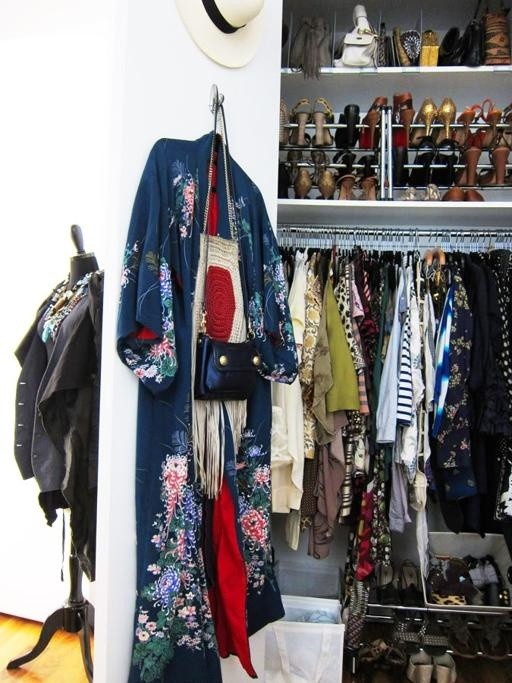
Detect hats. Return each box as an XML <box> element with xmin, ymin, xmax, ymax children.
<box><xmin>174</xmin><ymin>1</ymin><xmax>270</xmax><ymax>70</ymax></box>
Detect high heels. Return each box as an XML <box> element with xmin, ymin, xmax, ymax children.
<box><xmin>409</xmin><ymin>97</ymin><xmax>456</xmax><ymax>149</ymax></box>
<box><xmin>393</xmin><ymin>145</ymin><xmax>410</xmax><ymax>187</ymax></box>
<box><xmin>451</xmin><ymin>100</ymin><xmax>502</xmax><ymax>149</ymax></box>
<box><xmin>288</xmin><ymin>96</ymin><xmax>334</xmax><ymax>148</ymax></box>
<box><xmin>335</xmin><ymin>173</ymin><xmax>378</xmax><ymax>199</ymax></box>
<box><xmin>293</xmin><ymin>168</ymin><xmax>335</xmax><ymax>199</ymax></box>
<box><xmin>453</xmin><ymin>132</ymin><xmax>511</xmax><ymax>187</ymax></box>
<box><xmin>406</xmin><ymin>652</ymin><xmax>458</xmax><ymax>683</ymax></box>
<box><xmin>356</xmin><ymin>637</ymin><xmax>406</xmax><ymax>683</ymax></box>
<box><xmin>359</xmin><ymin>92</ymin><xmax>416</xmax><ymax>152</ymax></box>
<box><xmin>408</xmin><ymin>136</ymin><xmax>459</xmax><ymax>188</ymax></box>
<box><xmin>334</xmin><ymin>103</ymin><xmax>360</xmax><ymax>148</ymax></box>
<box><xmin>333</xmin><ymin>150</ymin><xmax>378</xmax><ymax>185</ymax></box>
<box><xmin>287</xmin><ymin>151</ymin><xmax>331</xmax><ymax>186</ymax></box>
<box><xmin>279</xmin><ymin>161</ymin><xmax>291</xmax><ymax>199</ymax></box>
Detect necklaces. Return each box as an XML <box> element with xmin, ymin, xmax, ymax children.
<box><xmin>41</xmin><ymin>271</ymin><xmax>94</xmax><ymax>342</ymax></box>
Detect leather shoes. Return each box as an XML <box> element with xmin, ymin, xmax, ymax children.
<box><xmin>428</xmin><ymin>568</ymin><xmax>467</xmax><ymax>605</ymax></box>
<box><xmin>279</xmin><ymin>97</ymin><xmax>290</xmax><ymax>146</ymax></box>
<box><xmin>375</xmin><ymin>558</ymin><xmax>424</xmax><ymax>607</ymax></box>
<box><xmin>463</xmin><ymin>554</ymin><xmax>510</xmax><ymax>607</ymax></box>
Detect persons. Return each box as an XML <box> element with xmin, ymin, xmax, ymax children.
<box><xmin>11</xmin><ymin>250</ymin><xmax>104</xmax><ymax>584</ymax></box>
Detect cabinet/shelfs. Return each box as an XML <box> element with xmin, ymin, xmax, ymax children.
<box><xmin>266</xmin><ymin>0</ymin><xmax>509</xmax><ymax>622</ymax></box>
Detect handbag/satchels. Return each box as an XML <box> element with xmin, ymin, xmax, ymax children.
<box><xmin>288</xmin><ymin>13</ymin><xmax>336</xmax><ymax>81</ymax></box>
<box><xmin>193</xmin><ymin>332</ymin><xmax>262</xmax><ymax>402</ymax></box>
<box><xmin>340</xmin><ymin>26</ymin><xmax>380</xmax><ymax>69</ymax></box>
<box><xmin>436</xmin><ymin>0</ymin><xmax>512</xmax><ymax>68</ymax></box>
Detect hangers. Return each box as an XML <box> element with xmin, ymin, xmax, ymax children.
<box><xmin>277</xmin><ymin>221</ymin><xmax>512</xmax><ymax>266</ymax></box>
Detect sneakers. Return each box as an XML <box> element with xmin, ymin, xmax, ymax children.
<box><xmin>442</xmin><ymin>187</ymin><xmax>485</xmax><ymax>201</ymax></box>
<box><xmin>397</xmin><ymin>183</ymin><xmax>441</xmax><ymax>200</ymax></box>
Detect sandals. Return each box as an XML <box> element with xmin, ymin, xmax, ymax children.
<box><xmin>448</xmin><ymin>613</ymin><xmax>509</xmax><ymax>660</ymax></box>
<box><xmin>497</xmin><ymin>103</ymin><xmax>512</xmax><ymax>148</ymax></box>
<box><xmin>391</xmin><ymin>609</ymin><xmax>449</xmax><ymax>656</ymax></box>
